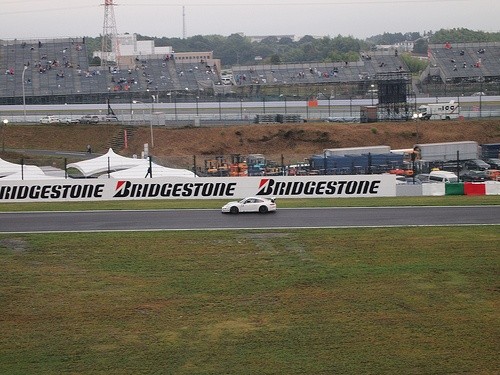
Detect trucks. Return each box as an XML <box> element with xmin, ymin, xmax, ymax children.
<box><xmin>418</xmin><ymin>103</ymin><xmax>460</xmax><ymax>121</ymax></box>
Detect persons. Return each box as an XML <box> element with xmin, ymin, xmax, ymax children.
<box><xmin>0</xmin><ymin>34</ymin><xmax>500</xmax><ymax>91</ymax></box>
<box><xmin>86</xmin><ymin>144</ymin><xmax>92</xmax><ymax>153</ymax></box>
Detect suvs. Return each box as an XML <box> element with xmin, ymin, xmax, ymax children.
<box><xmin>81</xmin><ymin>115</ymin><xmax>99</xmax><ymax>124</ymax></box>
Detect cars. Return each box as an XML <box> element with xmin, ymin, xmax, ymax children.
<box><xmin>221</xmin><ymin>197</ymin><xmax>276</xmax><ymax>214</ymax></box>
<box><xmin>222</xmin><ymin>78</ymin><xmax>230</xmax><ymax>85</ymax></box>
<box><xmin>60</xmin><ymin>117</ymin><xmax>79</xmax><ymax>125</ymax></box>
<box><xmin>202</xmin><ymin>145</ymin><xmax>500</xmax><ymax>188</ymax></box>
<box><xmin>39</xmin><ymin>116</ymin><xmax>59</xmax><ymax>125</ymax></box>
